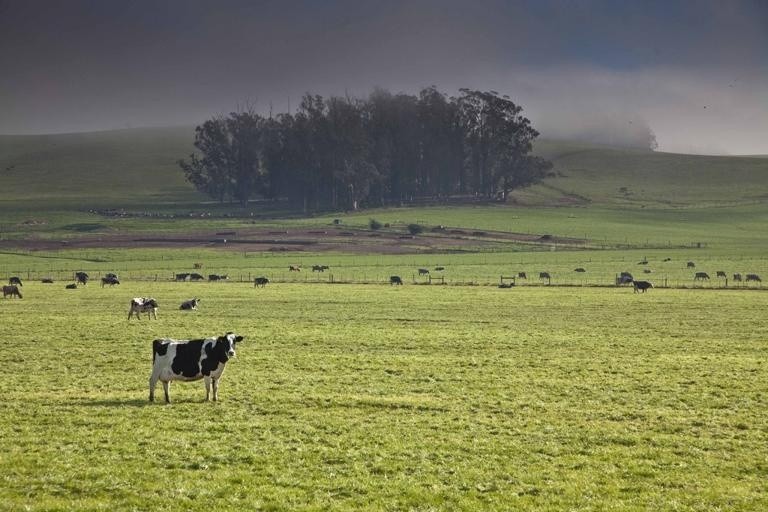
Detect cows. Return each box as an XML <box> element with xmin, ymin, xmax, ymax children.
<box><xmin>3</xmin><ymin>277</ymin><xmax>23</xmax><ymax>298</ymax></box>
<box><xmin>518</xmin><ymin>272</ymin><xmax>526</xmax><ymax>279</ymax></box>
<box><xmin>127</xmin><ymin>297</ymin><xmax>158</xmax><ymax>321</ymax></box>
<box><xmin>539</xmin><ymin>273</ymin><xmax>550</xmax><ymax>280</ymax></box>
<box><xmin>65</xmin><ymin>272</ymin><xmax>89</xmax><ymax>289</ymax></box>
<box><xmin>616</xmin><ymin>272</ymin><xmax>653</xmax><ymax>293</ymax></box>
<box><xmin>99</xmin><ymin>273</ymin><xmax>120</xmax><ymax>288</ymax></box>
<box><xmin>418</xmin><ymin>269</ymin><xmax>430</xmax><ymax>276</ymax></box>
<box><xmin>686</xmin><ymin>260</ymin><xmax>763</xmax><ymax>282</ymax></box>
<box><xmin>175</xmin><ymin>272</ymin><xmax>230</xmax><ymax>283</ymax></box>
<box><xmin>148</xmin><ymin>332</ymin><xmax>244</xmax><ymax>403</ymax></box>
<box><xmin>312</xmin><ymin>264</ymin><xmax>329</xmax><ymax>273</ymax></box>
<box><xmin>179</xmin><ymin>298</ymin><xmax>200</xmax><ymax>310</ymax></box>
<box><xmin>390</xmin><ymin>275</ymin><xmax>403</xmax><ymax>285</ymax></box>
<box><xmin>288</xmin><ymin>265</ymin><xmax>301</xmax><ymax>272</ymax></box>
<box><xmin>253</xmin><ymin>277</ymin><xmax>270</xmax><ymax>289</ymax></box>
